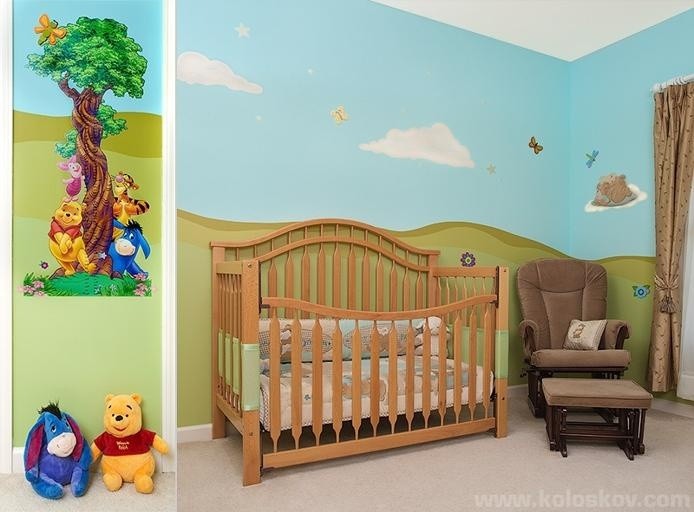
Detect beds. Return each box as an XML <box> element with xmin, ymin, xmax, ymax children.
<box><xmin>206</xmin><ymin>218</ymin><xmax>512</xmax><ymax>490</ymax></box>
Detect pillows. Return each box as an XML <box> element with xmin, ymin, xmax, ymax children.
<box><xmin>562</xmin><ymin>317</ymin><xmax>608</xmax><ymax>351</ymax></box>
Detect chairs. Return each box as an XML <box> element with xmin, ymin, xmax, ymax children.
<box><xmin>513</xmin><ymin>257</ymin><xmax>657</xmax><ymax>461</ymax></box>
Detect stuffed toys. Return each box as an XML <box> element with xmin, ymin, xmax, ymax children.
<box><xmin>22</xmin><ymin>398</ymin><xmax>92</xmax><ymax>502</ymax></box>
<box><xmin>91</xmin><ymin>394</ymin><xmax>172</xmax><ymax>495</ymax></box>
